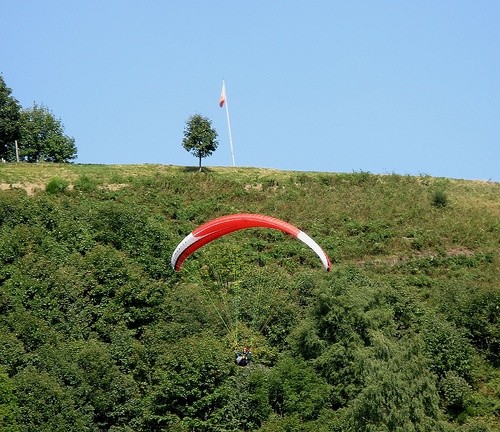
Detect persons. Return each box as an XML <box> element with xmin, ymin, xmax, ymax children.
<box><xmin>240</xmin><ymin>348</ymin><xmax>249</xmax><ymax>360</ymax></box>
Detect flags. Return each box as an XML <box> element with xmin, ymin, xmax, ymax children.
<box><xmin>219</xmin><ymin>89</ymin><xmax>225</xmax><ymax>108</ymax></box>
